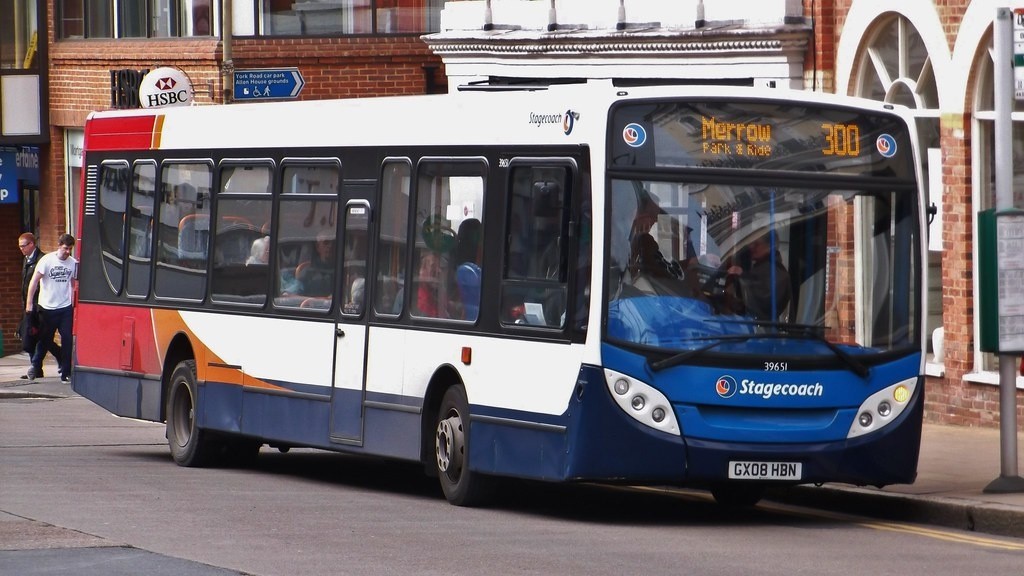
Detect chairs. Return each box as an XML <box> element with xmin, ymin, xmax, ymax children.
<box><xmin>137</xmin><ymin>212</ymin><xmax>517</xmax><ymax>321</ymax></box>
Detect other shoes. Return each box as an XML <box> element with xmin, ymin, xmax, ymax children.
<box><xmin>57</xmin><ymin>360</ymin><xmax>62</xmax><ymax>373</ymax></box>
<box><xmin>61</xmin><ymin>374</ymin><xmax>70</xmax><ymax>383</ymax></box>
<box><xmin>20</xmin><ymin>367</ymin><xmax>44</xmax><ymax>379</ymax></box>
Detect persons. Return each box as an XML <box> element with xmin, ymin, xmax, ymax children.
<box><xmin>727</xmin><ymin>228</ymin><xmax>791</xmax><ymax>321</ymax></box>
<box><xmin>26</xmin><ymin>234</ymin><xmax>79</xmax><ymax>384</ymax></box>
<box><xmin>417</xmin><ymin>215</ymin><xmax>481</xmax><ymax>321</ymax></box>
<box><xmin>18</xmin><ymin>232</ymin><xmax>62</xmax><ymax>379</ymax></box>
<box><xmin>302</xmin><ymin>227</ymin><xmax>336</xmax><ymax>297</ymax></box>
<box><xmin>633</xmin><ymin>192</ymin><xmax>699</xmax><ymax>277</ymax></box>
<box><xmin>250</xmin><ymin>220</ymin><xmax>283</xmax><ymax>264</ymax></box>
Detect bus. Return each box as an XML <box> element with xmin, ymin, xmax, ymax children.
<box><xmin>69</xmin><ymin>76</ymin><xmax>937</xmax><ymax>515</ymax></box>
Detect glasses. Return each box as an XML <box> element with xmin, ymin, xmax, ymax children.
<box><xmin>19</xmin><ymin>242</ymin><xmax>30</xmax><ymax>249</ymax></box>
